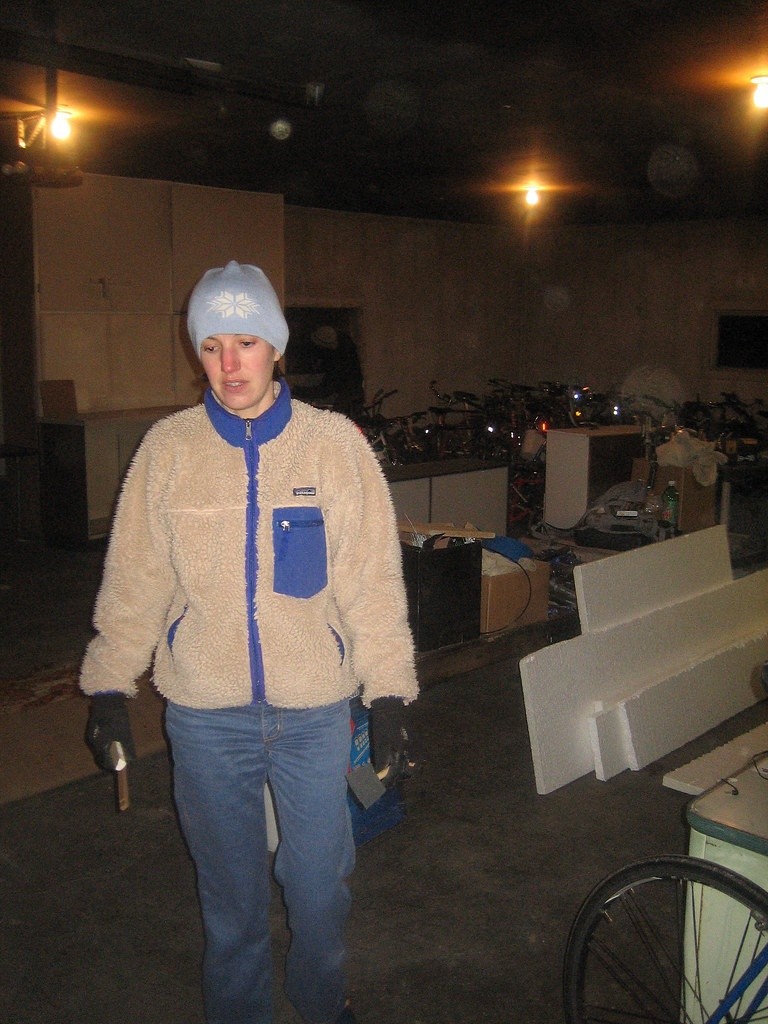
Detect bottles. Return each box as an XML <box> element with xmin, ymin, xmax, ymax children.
<box><xmin>663</xmin><ymin>480</ymin><xmax>678</xmax><ymax>523</ymax></box>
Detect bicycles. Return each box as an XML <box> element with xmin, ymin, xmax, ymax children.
<box><xmin>310</xmin><ymin>377</ymin><xmax>768</xmax><ymax>529</ymax></box>
<box><xmin>562</xmin><ymin>855</ymin><xmax>768</xmax><ymax>1024</ymax></box>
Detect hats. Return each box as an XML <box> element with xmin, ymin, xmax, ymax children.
<box><xmin>186</xmin><ymin>260</ymin><xmax>289</xmax><ymax>359</ymax></box>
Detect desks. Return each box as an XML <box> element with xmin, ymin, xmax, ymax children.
<box><xmin>42</xmin><ymin>405</ymin><xmax>197</xmax><ymax>545</ymax></box>
<box><xmin>373</xmin><ymin>458</ymin><xmax>508</xmax><ymax>541</ymax></box>
<box><xmin>544</xmin><ymin>425</ymin><xmax>657</xmax><ymax>528</ymax></box>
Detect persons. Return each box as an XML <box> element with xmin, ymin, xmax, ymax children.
<box><xmin>80</xmin><ymin>258</ymin><xmax>421</xmax><ymax>1024</ymax></box>
<box><xmin>290</xmin><ymin>326</ymin><xmax>366</xmax><ymax>415</ymax></box>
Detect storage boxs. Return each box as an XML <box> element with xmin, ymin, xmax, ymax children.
<box><xmin>480</xmin><ymin>554</ymin><xmax>551</xmax><ymax>636</ymax></box>
<box><xmin>630</xmin><ymin>455</ymin><xmax>717</xmax><ymax>533</ymax></box>
<box><xmin>399</xmin><ymin>518</ymin><xmax>495</xmax><ymax>651</ymax></box>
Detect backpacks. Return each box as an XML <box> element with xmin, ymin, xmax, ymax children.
<box><xmin>535</xmin><ymin>480</ymin><xmax>671</xmax><ymax>552</ymax></box>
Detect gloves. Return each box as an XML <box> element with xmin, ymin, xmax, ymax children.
<box><xmin>85</xmin><ymin>691</ymin><xmax>137</xmax><ymax>772</ymax></box>
<box><xmin>369</xmin><ymin>696</ymin><xmax>415</xmax><ymax>779</ymax></box>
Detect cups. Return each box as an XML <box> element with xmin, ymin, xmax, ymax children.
<box><xmin>726</xmin><ymin>440</ymin><xmax>736</xmax><ymax>454</ymax></box>
